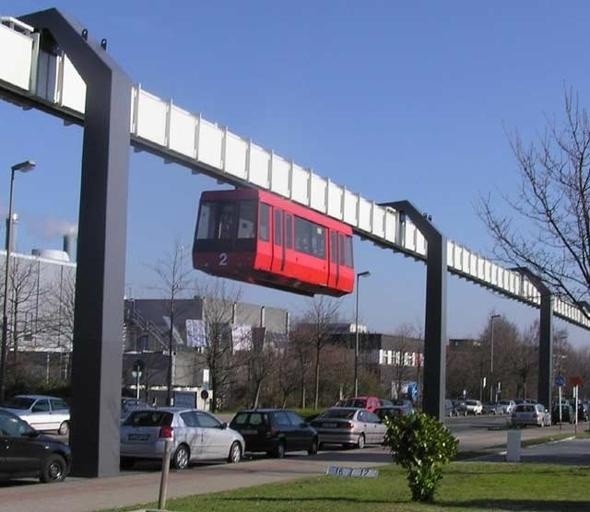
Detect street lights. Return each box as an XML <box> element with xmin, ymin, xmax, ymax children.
<box><xmin>2</xmin><ymin>160</ymin><xmax>35</xmax><ymax>398</ymax></box>
<box><xmin>356</xmin><ymin>272</ymin><xmax>370</xmax><ymax>396</ymax></box>
<box><xmin>489</xmin><ymin>314</ymin><xmax>501</xmax><ymax>403</ymax></box>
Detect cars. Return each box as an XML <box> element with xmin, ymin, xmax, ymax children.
<box><xmin>0</xmin><ymin>394</ymin><xmax>70</xmax><ymax>436</ymax></box>
<box><xmin>311</xmin><ymin>398</ymin><xmax>413</xmax><ymax>449</ymax></box>
<box><xmin>228</xmin><ymin>409</ymin><xmax>320</xmax><ymax>459</ymax></box>
<box><xmin>0</xmin><ymin>409</ymin><xmax>72</xmax><ymax>484</ymax></box>
<box><xmin>444</xmin><ymin>398</ymin><xmax>589</xmax><ymax>428</ymax></box>
<box><xmin>120</xmin><ymin>407</ymin><xmax>245</xmax><ymax>468</ymax></box>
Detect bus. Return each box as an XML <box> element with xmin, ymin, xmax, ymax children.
<box><xmin>191</xmin><ymin>188</ymin><xmax>354</xmax><ymax>297</ymax></box>
